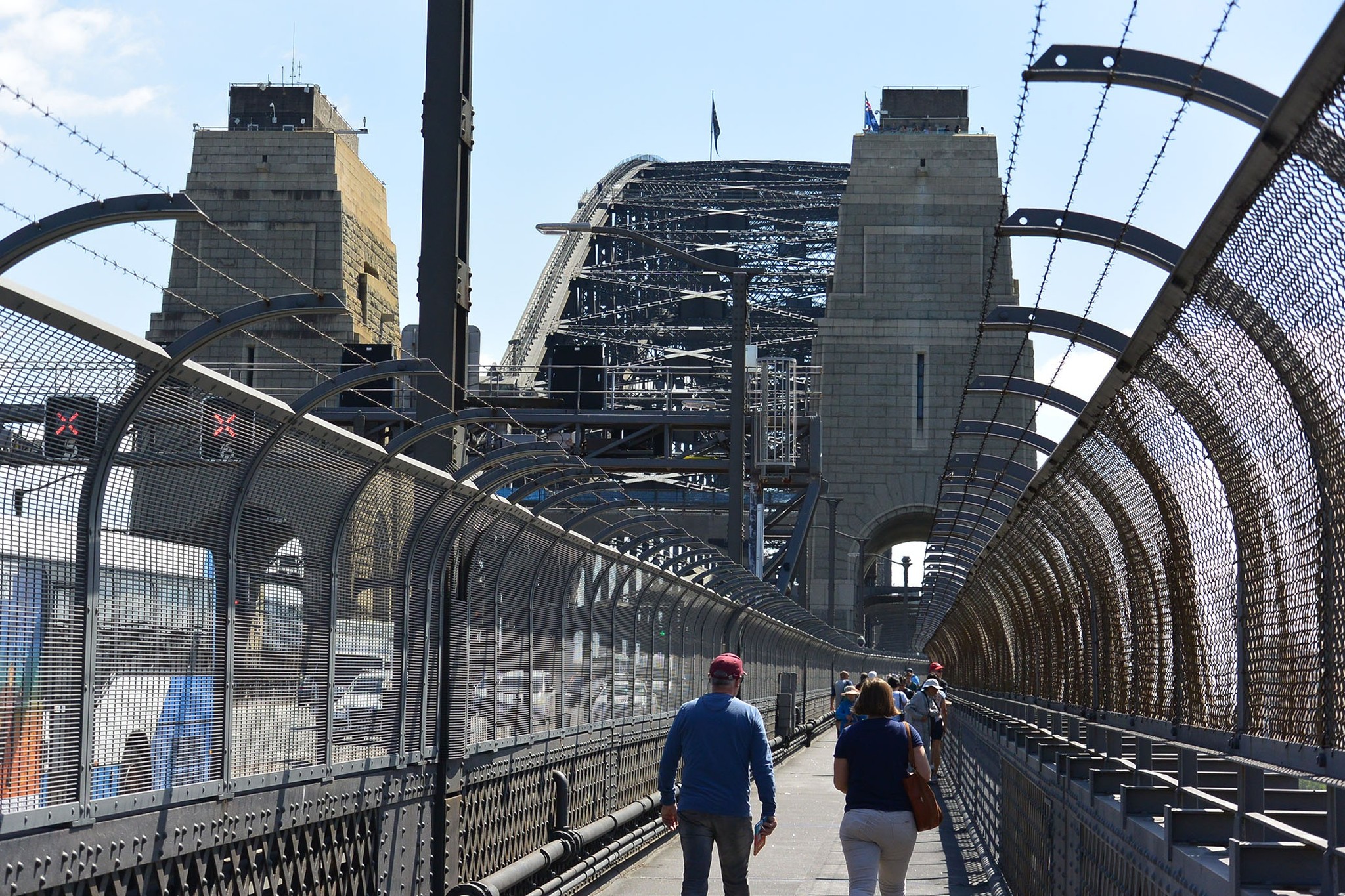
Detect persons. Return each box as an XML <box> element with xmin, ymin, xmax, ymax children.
<box><xmin>657</xmin><ymin>652</ymin><xmax>777</xmax><ymax>896</ymax></box>
<box><xmin>878</xmin><ymin>122</ymin><xmax>988</xmax><ymax>135</ymax></box>
<box><xmin>833</xmin><ymin>676</ymin><xmax>932</xmax><ymax>896</ymax></box>
<box><xmin>830</xmin><ymin>662</ymin><xmax>952</xmax><ymax>785</ymax></box>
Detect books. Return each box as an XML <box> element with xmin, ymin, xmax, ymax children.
<box><xmin>754</xmin><ymin>815</ymin><xmax>765</xmax><ymax>856</ymax></box>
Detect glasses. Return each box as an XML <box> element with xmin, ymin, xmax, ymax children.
<box><xmin>936</xmin><ymin>671</ymin><xmax>945</xmax><ymax>673</ymax></box>
<box><xmin>735</xmin><ymin>675</ymin><xmax>744</xmax><ymax>683</ymax></box>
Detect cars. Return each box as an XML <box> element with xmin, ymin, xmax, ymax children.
<box><xmin>297</xmin><ymin>676</ymin><xmax>350</xmax><ymax>708</ymax></box>
<box><xmin>470</xmin><ymin>675</ymin><xmax>503</xmax><ymax>716</ymax></box>
<box><xmin>564</xmin><ymin>668</ymin><xmax>673</xmax><ymax>722</ymax></box>
<box><xmin>494</xmin><ymin>670</ymin><xmax>556</xmax><ymax>725</ymax></box>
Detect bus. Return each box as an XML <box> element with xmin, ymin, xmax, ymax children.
<box><xmin>0</xmin><ymin>512</ymin><xmax>216</xmax><ymax>813</ymax></box>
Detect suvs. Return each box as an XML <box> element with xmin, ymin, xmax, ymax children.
<box><xmin>331</xmin><ymin>670</ymin><xmax>392</xmax><ymax>744</ymax></box>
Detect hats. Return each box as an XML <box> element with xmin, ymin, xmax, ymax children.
<box><xmin>709</xmin><ymin>653</ymin><xmax>748</xmax><ymax>681</ymax></box>
<box><xmin>922</xmin><ymin>678</ymin><xmax>943</xmax><ymax>689</ymax></box>
<box><xmin>929</xmin><ymin>663</ymin><xmax>945</xmax><ymax>671</ymax></box>
<box><xmin>868</xmin><ymin>671</ymin><xmax>877</xmax><ymax>678</ymax></box>
<box><xmin>840</xmin><ymin>686</ymin><xmax>861</xmax><ymax>696</ymax></box>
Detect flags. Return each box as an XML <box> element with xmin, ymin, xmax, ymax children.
<box><xmin>713</xmin><ymin>99</ymin><xmax>721</xmax><ymax>157</ymax></box>
<box><xmin>865</xmin><ymin>96</ymin><xmax>879</xmax><ymax>132</ymax></box>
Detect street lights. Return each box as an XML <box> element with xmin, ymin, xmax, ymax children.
<box><xmin>846</xmin><ymin>552</ymin><xmax>913</xmax><ymax>655</ymax></box>
<box><xmin>535</xmin><ymin>222</ymin><xmax>768</xmax><ymax>568</ymax></box>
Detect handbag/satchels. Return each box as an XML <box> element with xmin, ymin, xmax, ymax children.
<box><xmin>902</xmin><ymin>721</ymin><xmax>942</xmax><ymax>832</ymax></box>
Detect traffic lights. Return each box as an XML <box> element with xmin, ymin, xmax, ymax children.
<box><xmin>41</xmin><ymin>395</ymin><xmax>99</xmax><ymax>460</ymax></box>
<box><xmin>201</xmin><ymin>396</ymin><xmax>255</xmax><ymax>463</ymax></box>
